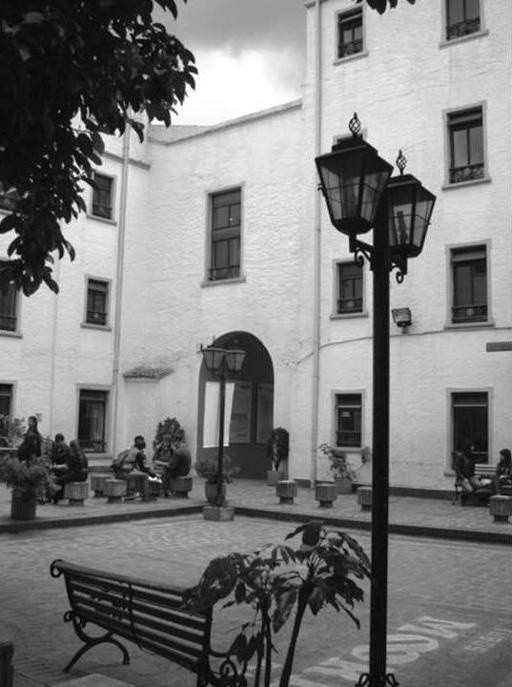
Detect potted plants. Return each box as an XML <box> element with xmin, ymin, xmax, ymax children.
<box><xmin>195</xmin><ymin>453</ymin><xmax>241</xmax><ymax>506</ymax></box>
<box><xmin>264</xmin><ymin>427</ymin><xmax>290</xmax><ymax>485</ymax></box>
<box><xmin>320</xmin><ymin>443</ymin><xmax>370</xmax><ymax>494</ymax></box>
<box><xmin>2</xmin><ymin>456</ymin><xmax>50</xmax><ymax>520</ymax></box>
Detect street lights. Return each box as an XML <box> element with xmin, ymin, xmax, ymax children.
<box><xmin>200</xmin><ymin>339</ymin><xmax>247</xmax><ymax>505</ymax></box>
<box><xmin>313</xmin><ymin>112</ymin><xmax>437</xmax><ymax>687</ymax></box>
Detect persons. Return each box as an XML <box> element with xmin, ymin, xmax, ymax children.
<box><xmin>495</xmin><ymin>449</ymin><xmax>512</xmax><ymax>506</ymax></box>
<box><xmin>19</xmin><ymin>417</ymin><xmax>88</xmax><ymax>505</ymax></box>
<box><xmin>455</xmin><ymin>440</ymin><xmax>483</xmax><ymax>506</ymax></box>
<box><xmin>113</xmin><ymin>434</ymin><xmax>191</xmax><ymax>498</ymax></box>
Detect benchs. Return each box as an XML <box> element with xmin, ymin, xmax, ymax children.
<box><xmin>49</xmin><ymin>553</ymin><xmax>251</xmax><ymax>687</ymax></box>
